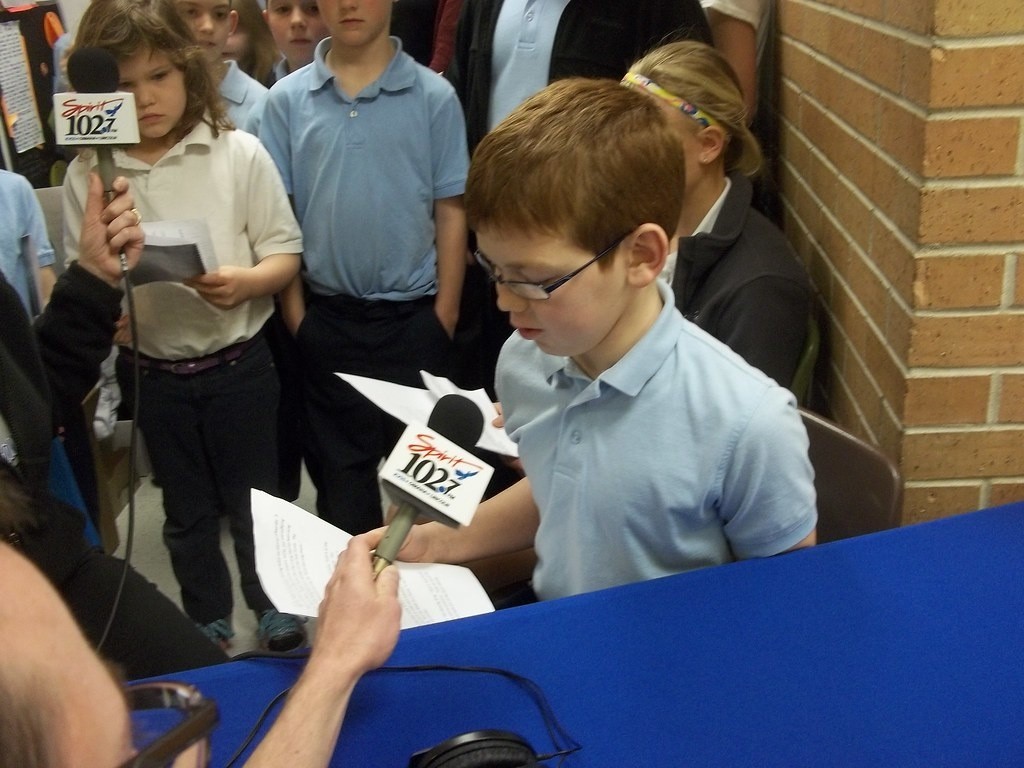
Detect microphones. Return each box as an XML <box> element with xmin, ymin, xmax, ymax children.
<box><xmin>364</xmin><ymin>393</ymin><xmax>494</xmax><ymax>583</ymax></box>
<box><xmin>52</xmin><ymin>46</ymin><xmax>142</xmax><ymax>209</ymax></box>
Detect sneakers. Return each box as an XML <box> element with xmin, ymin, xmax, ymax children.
<box><xmin>196</xmin><ymin>614</ymin><xmax>233</xmax><ymax>651</ymax></box>
<box><xmin>255</xmin><ymin>606</ymin><xmax>307</xmax><ymax>650</ymax></box>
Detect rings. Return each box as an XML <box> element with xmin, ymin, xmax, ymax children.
<box><xmin>131</xmin><ymin>208</ymin><xmax>142</xmax><ymax>226</ymax></box>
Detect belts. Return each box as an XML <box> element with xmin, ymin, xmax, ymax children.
<box><xmin>120</xmin><ymin>350</ymin><xmax>241</xmax><ymax>374</ymax></box>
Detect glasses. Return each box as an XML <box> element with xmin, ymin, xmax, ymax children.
<box><xmin>474</xmin><ymin>221</ymin><xmax>669</xmax><ymax>299</ymax></box>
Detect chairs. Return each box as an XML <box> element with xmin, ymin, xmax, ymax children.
<box><xmin>774</xmin><ymin>318</ymin><xmax>902</xmax><ymax>541</ymax></box>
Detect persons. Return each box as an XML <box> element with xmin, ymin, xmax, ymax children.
<box><xmin>445</xmin><ymin>0</ymin><xmax>761</xmax><ymax>404</ymax></box>
<box><xmin>173</xmin><ymin>0</ymin><xmax>330</xmax><ymax>502</ymax></box>
<box><xmin>63</xmin><ymin>0</ymin><xmax>304</xmax><ymax>650</ymax></box>
<box><xmin>375</xmin><ymin>77</ymin><xmax>818</xmax><ymax>599</ymax></box>
<box><xmin>618</xmin><ymin>41</ymin><xmax>810</xmax><ymax>390</ymax></box>
<box><xmin>0</xmin><ymin>535</ymin><xmax>403</xmax><ymax>768</ymax></box>
<box><xmin>0</xmin><ymin>169</ymin><xmax>56</xmax><ymax>318</ymax></box>
<box><xmin>259</xmin><ymin>0</ymin><xmax>471</xmax><ymax>536</ymax></box>
<box><xmin>0</xmin><ymin>173</ymin><xmax>230</xmax><ymax>680</ymax></box>
